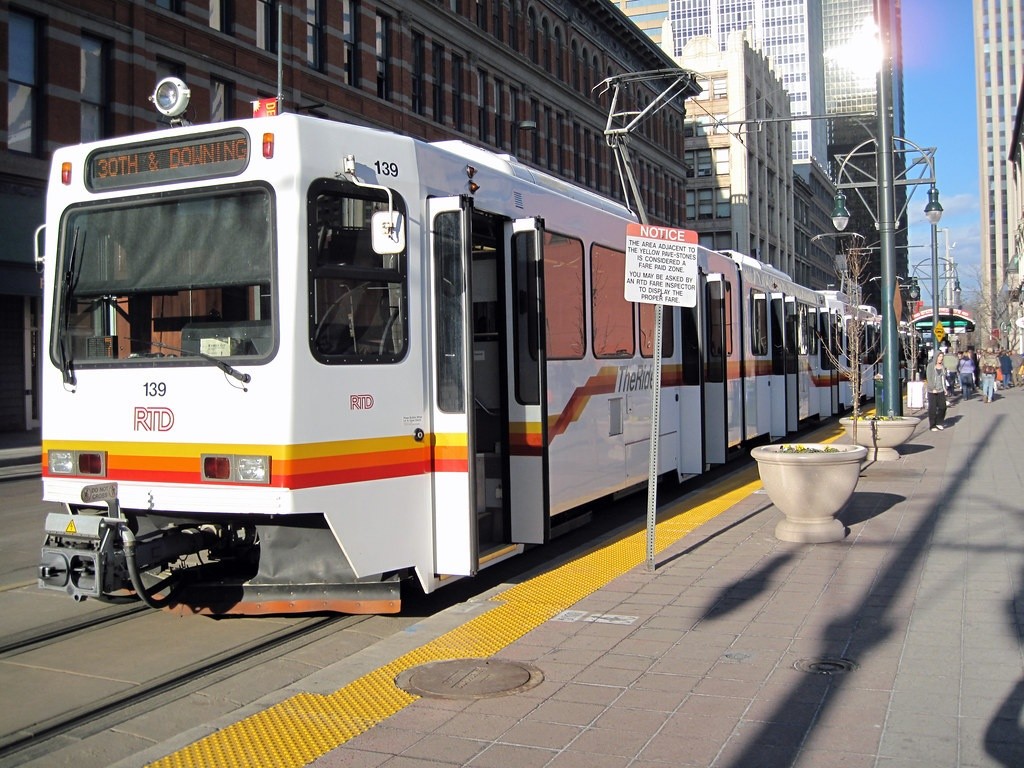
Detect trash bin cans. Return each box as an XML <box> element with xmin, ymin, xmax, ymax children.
<box><xmin>872</xmin><ymin>378</ymin><xmax>904</xmax><ymax>416</ymax></box>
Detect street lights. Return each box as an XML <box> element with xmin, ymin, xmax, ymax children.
<box><xmin>911</xmin><ymin>256</ymin><xmax>961</xmax><ymax>352</ymax></box>
<box><xmin>832</xmin><ymin>135</ymin><xmax>954</xmax><ymax>416</ymax></box>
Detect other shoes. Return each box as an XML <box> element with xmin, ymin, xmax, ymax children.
<box><xmin>936</xmin><ymin>425</ymin><xmax>944</xmax><ymax>430</ymax></box>
<box><xmin>931</xmin><ymin>427</ymin><xmax>938</xmax><ymax>432</ymax></box>
<box><xmin>988</xmin><ymin>399</ymin><xmax>992</xmax><ymax>403</ymax></box>
<box><xmin>983</xmin><ymin>396</ymin><xmax>987</xmax><ymax>402</ymax></box>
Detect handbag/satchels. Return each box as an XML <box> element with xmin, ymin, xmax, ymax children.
<box><xmin>995</xmin><ymin>368</ymin><xmax>1004</xmax><ymax>381</ymax></box>
<box><xmin>972</xmin><ymin>373</ymin><xmax>975</xmax><ymax>383</ymax></box>
<box><xmin>994</xmin><ymin>382</ymin><xmax>997</xmax><ymax>392</ymax></box>
<box><xmin>982</xmin><ymin>364</ymin><xmax>996</xmax><ymax>374</ymax></box>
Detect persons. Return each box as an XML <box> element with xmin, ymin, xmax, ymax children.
<box><xmin>921</xmin><ymin>346</ymin><xmax>1024</xmax><ymax>403</ymax></box>
<box><xmin>926</xmin><ymin>349</ymin><xmax>949</xmax><ymax>432</ymax></box>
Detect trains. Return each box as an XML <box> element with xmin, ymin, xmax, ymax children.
<box><xmin>27</xmin><ymin>116</ymin><xmax>923</xmax><ymax>616</ymax></box>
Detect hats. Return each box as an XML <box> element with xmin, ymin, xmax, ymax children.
<box><xmin>987</xmin><ymin>348</ymin><xmax>993</xmax><ymax>353</ymax></box>
